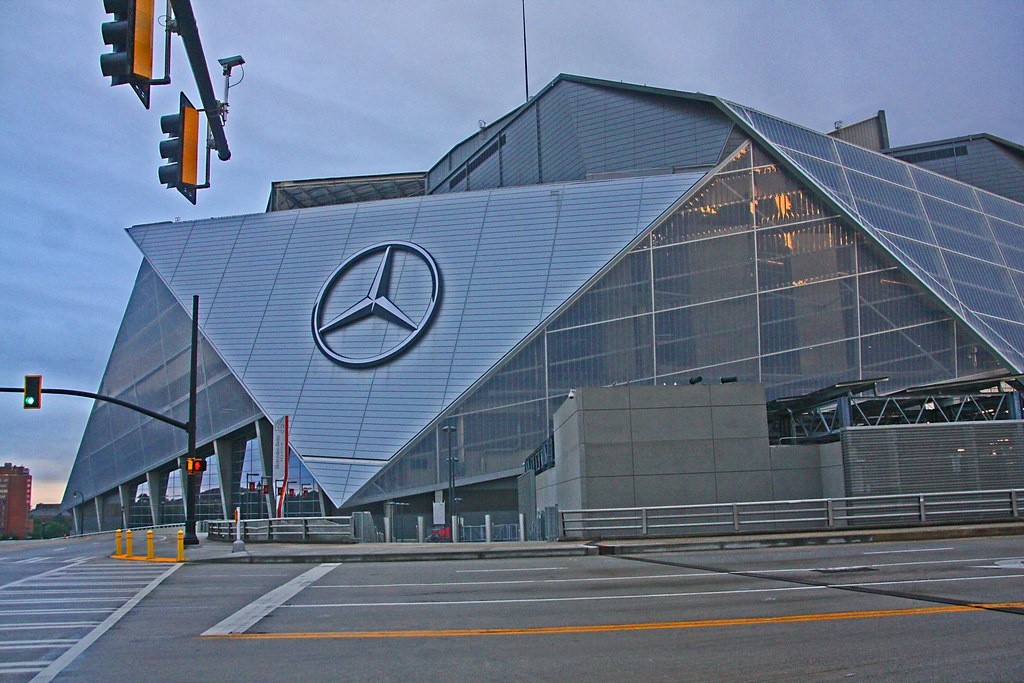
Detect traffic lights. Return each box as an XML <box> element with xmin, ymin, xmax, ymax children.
<box><xmin>23</xmin><ymin>374</ymin><xmax>42</xmax><ymax>409</ymax></box>
<box><xmin>187</xmin><ymin>458</ymin><xmax>207</xmax><ymax>473</ymax></box>
<box><xmin>158</xmin><ymin>92</ymin><xmax>199</xmax><ymax>207</ymax></box>
<box><xmin>100</xmin><ymin>0</ymin><xmax>155</xmax><ymax>109</ymax></box>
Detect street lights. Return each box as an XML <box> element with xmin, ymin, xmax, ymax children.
<box><xmin>74</xmin><ymin>490</ymin><xmax>84</xmax><ymax>537</ymax></box>
<box><xmin>442</xmin><ymin>425</ymin><xmax>457</xmax><ymax>539</ymax></box>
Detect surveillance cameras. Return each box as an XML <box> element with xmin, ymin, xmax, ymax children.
<box><xmin>569</xmin><ymin>393</ymin><xmax>574</xmax><ymax>398</ymax></box>
<box><xmin>217</xmin><ymin>55</ymin><xmax>245</xmax><ymax>66</ymax></box>
